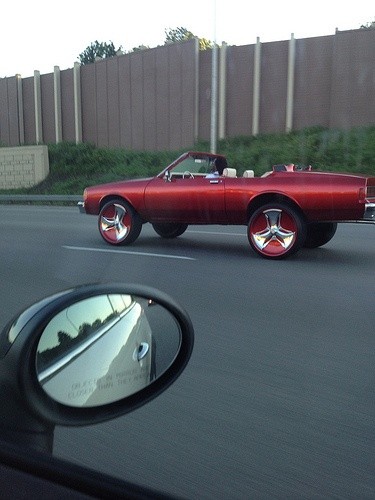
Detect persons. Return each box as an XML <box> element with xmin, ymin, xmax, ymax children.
<box><xmin>189</xmin><ymin>158</ymin><xmax>228</xmax><ymax>179</ymax></box>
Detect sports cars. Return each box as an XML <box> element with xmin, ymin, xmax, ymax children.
<box><xmin>78</xmin><ymin>150</ymin><xmax>375</xmax><ymax>261</ymax></box>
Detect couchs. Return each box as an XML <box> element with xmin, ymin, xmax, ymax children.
<box><xmin>218</xmin><ymin>168</ymin><xmax>274</xmax><ymax>178</ymax></box>
